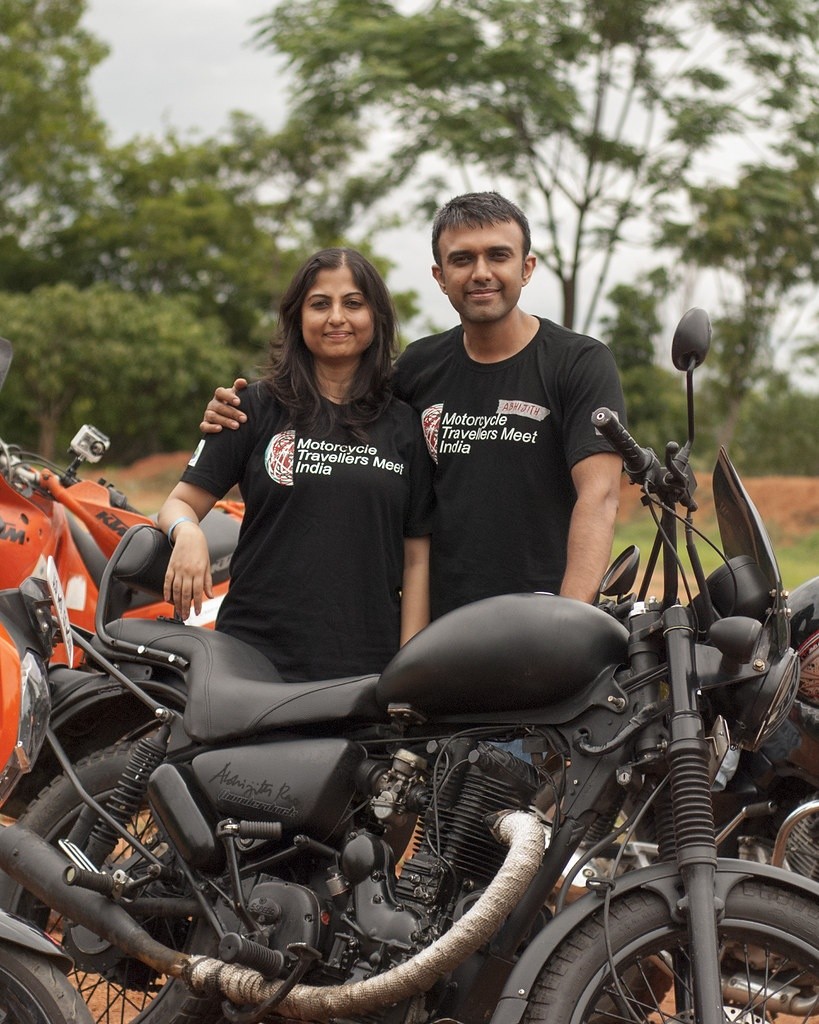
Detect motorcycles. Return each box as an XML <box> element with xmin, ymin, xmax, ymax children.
<box><xmin>1</xmin><ymin>301</ymin><xmax>818</xmax><ymax>1024</ymax></box>
<box><xmin>58</xmin><ymin>340</ymin><xmax>251</xmax><ymax>825</ymax></box>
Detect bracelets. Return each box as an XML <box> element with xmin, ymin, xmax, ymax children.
<box><xmin>168</xmin><ymin>517</ymin><xmax>193</xmax><ymax>550</ymax></box>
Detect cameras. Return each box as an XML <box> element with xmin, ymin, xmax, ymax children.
<box><xmin>70</xmin><ymin>423</ymin><xmax>111</xmax><ymax>464</ymax></box>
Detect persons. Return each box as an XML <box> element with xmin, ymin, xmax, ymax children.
<box><xmin>199</xmin><ymin>194</ymin><xmax>628</xmax><ymax>907</ymax></box>
<box><xmin>157</xmin><ymin>248</ymin><xmax>438</xmax><ymax>686</ymax></box>
